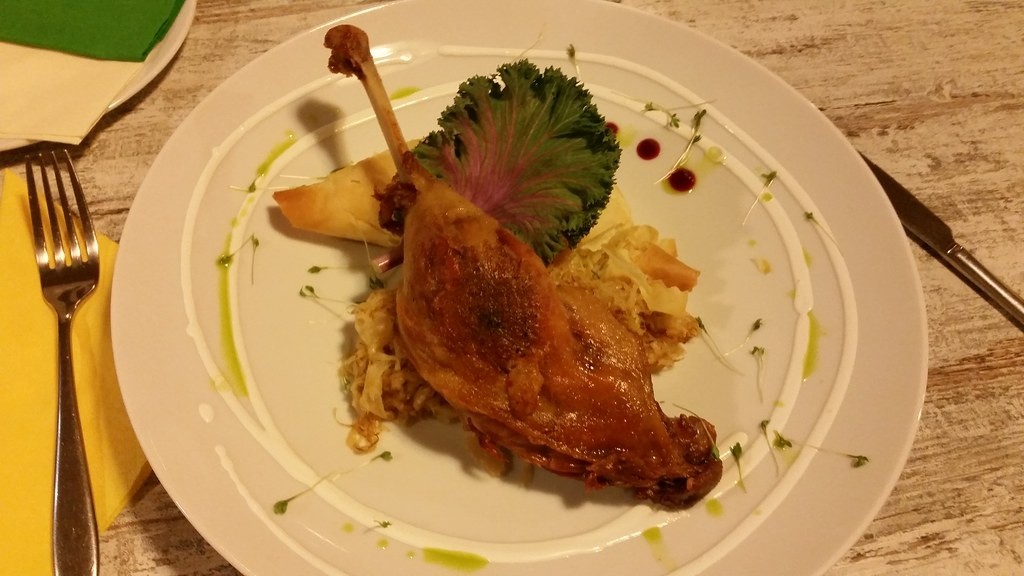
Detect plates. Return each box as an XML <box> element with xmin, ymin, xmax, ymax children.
<box><xmin>111</xmin><ymin>0</ymin><xmax>931</xmax><ymax>576</ymax></box>
<box><xmin>103</xmin><ymin>0</ymin><xmax>200</xmax><ymax>123</ymax></box>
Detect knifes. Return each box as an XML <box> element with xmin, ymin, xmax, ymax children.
<box><xmin>855</xmin><ymin>149</ymin><xmax>1024</xmax><ymax>326</ymax></box>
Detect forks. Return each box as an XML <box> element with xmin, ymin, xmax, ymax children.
<box><xmin>23</xmin><ymin>149</ymin><xmax>101</xmax><ymax>576</ymax></box>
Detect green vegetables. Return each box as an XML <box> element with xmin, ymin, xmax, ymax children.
<box><xmin>411</xmin><ymin>59</ymin><xmax>623</xmax><ymax>266</ymax></box>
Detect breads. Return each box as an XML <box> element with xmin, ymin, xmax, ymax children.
<box><xmin>274</xmin><ymin>136</ymin><xmax>628</xmax><ymax>249</ymax></box>
